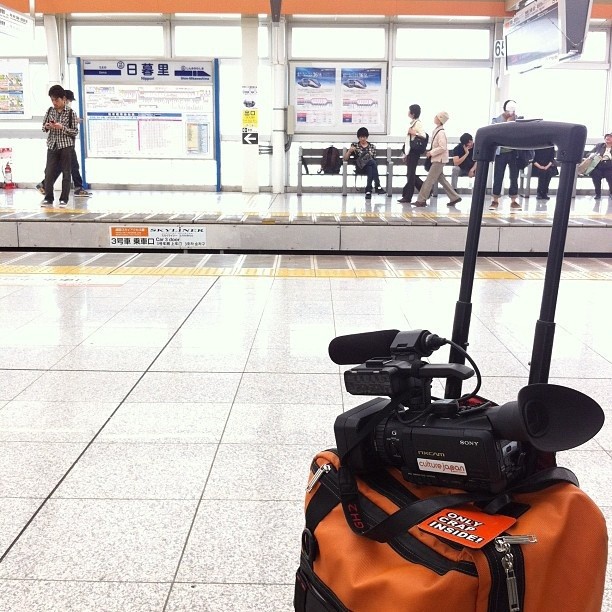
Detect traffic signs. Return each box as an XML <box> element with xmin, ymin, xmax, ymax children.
<box><xmin>241</xmin><ymin>109</ymin><xmax>258</xmax><ymax>145</ymax></box>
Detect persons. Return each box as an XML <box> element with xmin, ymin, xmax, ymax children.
<box><xmin>397</xmin><ymin>105</ymin><xmax>433</xmax><ymax>202</ymax></box>
<box><xmin>40</xmin><ymin>86</ymin><xmax>78</xmax><ymax>208</ymax></box>
<box><xmin>414</xmin><ymin>112</ymin><xmax>462</xmax><ymax>207</ymax></box>
<box><xmin>488</xmin><ymin>100</ymin><xmax>524</xmax><ymax>210</ymax></box>
<box><xmin>452</xmin><ymin>133</ymin><xmax>477</xmax><ymax>194</ymax></box>
<box><xmin>344</xmin><ymin>127</ymin><xmax>387</xmax><ymax>199</ymax></box>
<box><xmin>531</xmin><ymin>147</ymin><xmax>558</xmax><ymax>199</ymax></box>
<box><xmin>35</xmin><ymin>90</ymin><xmax>93</xmax><ymax>197</ymax></box>
<box><xmin>585</xmin><ymin>132</ymin><xmax>611</xmax><ymax>199</ymax></box>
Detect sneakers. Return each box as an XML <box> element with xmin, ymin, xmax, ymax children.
<box><xmin>36</xmin><ymin>183</ymin><xmax>46</xmax><ymax>195</ymax></box>
<box><xmin>377</xmin><ymin>187</ymin><xmax>386</xmax><ymax>195</ymax></box>
<box><xmin>58</xmin><ymin>201</ymin><xmax>69</xmax><ymax>207</ymax></box>
<box><xmin>74</xmin><ymin>187</ymin><xmax>92</xmax><ymax>197</ymax></box>
<box><xmin>488</xmin><ymin>201</ymin><xmax>499</xmax><ymax>209</ymax></box>
<box><xmin>365</xmin><ymin>192</ymin><xmax>371</xmax><ymax>199</ymax></box>
<box><xmin>40</xmin><ymin>199</ymin><xmax>53</xmax><ymax>206</ymax></box>
<box><xmin>510</xmin><ymin>202</ymin><xmax>521</xmax><ymax>209</ymax></box>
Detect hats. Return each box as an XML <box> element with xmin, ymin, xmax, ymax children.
<box><xmin>604</xmin><ymin>132</ymin><xmax>612</xmax><ymax>139</ymax></box>
<box><xmin>503</xmin><ymin>100</ymin><xmax>516</xmax><ymax>114</ymax></box>
<box><xmin>63</xmin><ymin>90</ymin><xmax>76</xmax><ymax>101</ymax></box>
<box><xmin>436</xmin><ymin>111</ymin><xmax>449</xmax><ymax>124</ymax></box>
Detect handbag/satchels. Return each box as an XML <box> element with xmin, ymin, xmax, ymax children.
<box><xmin>355</xmin><ymin>146</ymin><xmax>373</xmax><ymax>169</ymax></box>
<box><xmin>409</xmin><ymin>120</ymin><xmax>429</xmax><ymax>154</ymax></box>
<box><xmin>424</xmin><ymin>129</ymin><xmax>445</xmax><ymax>171</ymax></box>
<box><xmin>578</xmin><ymin>141</ymin><xmax>602</xmax><ymax>175</ymax></box>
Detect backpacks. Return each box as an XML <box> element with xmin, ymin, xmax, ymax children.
<box><xmin>317</xmin><ymin>147</ymin><xmax>340</xmax><ymax>174</ymax></box>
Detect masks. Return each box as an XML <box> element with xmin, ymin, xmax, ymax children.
<box><xmin>65</xmin><ymin>101</ymin><xmax>72</xmax><ymax>107</ymax></box>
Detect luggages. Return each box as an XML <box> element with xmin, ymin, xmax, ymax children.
<box><xmin>293</xmin><ymin>118</ymin><xmax>607</xmax><ymax>612</ymax></box>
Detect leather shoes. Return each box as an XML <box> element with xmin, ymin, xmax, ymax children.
<box><xmin>411</xmin><ymin>201</ymin><xmax>426</xmax><ymax>207</ymax></box>
<box><xmin>593</xmin><ymin>193</ymin><xmax>601</xmax><ymax>199</ymax></box>
<box><xmin>447</xmin><ymin>197</ymin><xmax>461</xmax><ymax>206</ymax></box>
<box><xmin>397</xmin><ymin>196</ymin><xmax>411</xmax><ymax>203</ymax></box>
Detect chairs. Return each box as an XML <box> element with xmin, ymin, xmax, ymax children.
<box><xmin>345</xmin><ymin>148</ymin><xmax>391</xmax><ymax>197</ymax></box>
<box><xmin>298</xmin><ymin>147</ymin><xmax>345</xmax><ymax>195</ymax></box>
<box><xmin>436</xmin><ymin>149</ymin><xmax>482</xmax><ymax>197</ymax></box>
<box><xmin>390</xmin><ymin>149</ymin><xmax>436</xmax><ymax>198</ymax></box>
<box><xmin>517</xmin><ymin>149</ymin><xmax>558</xmax><ymax>197</ymax></box>
<box><xmin>571</xmin><ymin>151</ymin><xmax>604</xmax><ymax>199</ymax></box>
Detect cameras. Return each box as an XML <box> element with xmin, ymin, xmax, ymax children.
<box><xmin>325</xmin><ymin>326</ymin><xmax>607</xmax><ymax>517</ymax></box>
